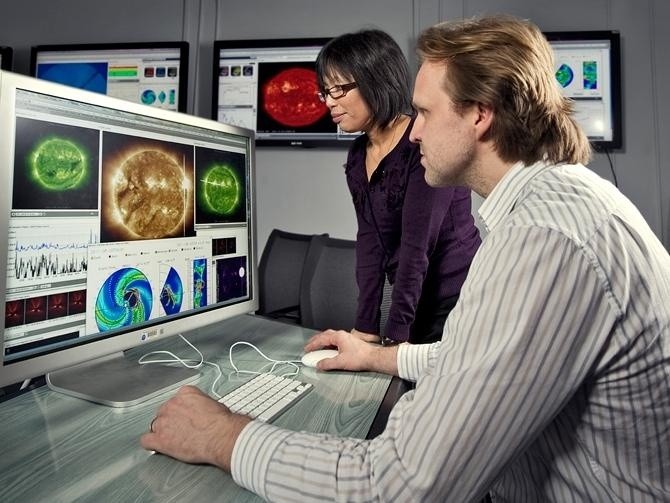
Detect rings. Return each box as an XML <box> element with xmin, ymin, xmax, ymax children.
<box><xmin>149</xmin><ymin>416</ymin><xmax>157</xmax><ymax>431</ymax></box>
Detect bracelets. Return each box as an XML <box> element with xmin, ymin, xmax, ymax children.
<box><xmin>378</xmin><ymin>335</ymin><xmax>398</xmax><ymax>347</ymax></box>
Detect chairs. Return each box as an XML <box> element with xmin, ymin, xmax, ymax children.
<box><xmin>255</xmin><ymin>228</ymin><xmax>357</xmax><ymax>329</ymax></box>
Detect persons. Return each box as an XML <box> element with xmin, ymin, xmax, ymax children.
<box><xmin>312</xmin><ymin>25</ymin><xmax>485</xmax><ymax>391</ymax></box>
<box><xmin>136</xmin><ymin>9</ymin><xmax>669</xmax><ymax>502</ymax></box>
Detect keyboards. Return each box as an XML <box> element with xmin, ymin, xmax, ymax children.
<box><xmin>214</xmin><ymin>370</ymin><xmax>314</xmax><ymax>426</ymax></box>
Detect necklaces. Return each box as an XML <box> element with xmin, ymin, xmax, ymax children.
<box><xmin>369</xmin><ymin>130</ymin><xmax>397</xmax><ymax>167</ymax></box>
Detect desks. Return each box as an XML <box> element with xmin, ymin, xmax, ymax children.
<box><xmin>0</xmin><ymin>312</ymin><xmax>395</xmax><ymax>502</ymax></box>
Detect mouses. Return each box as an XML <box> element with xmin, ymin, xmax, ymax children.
<box><xmin>301</xmin><ymin>348</ymin><xmax>338</xmax><ymax>369</ymax></box>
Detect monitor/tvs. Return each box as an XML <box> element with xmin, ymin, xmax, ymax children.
<box><xmin>211</xmin><ymin>38</ymin><xmax>364</xmax><ymax>151</ymax></box>
<box><xmin>542</xmin><ymin>30</ymin><xmax>622</xmax><ymax>152</ymax></box>
<box><xmin>1</xmin><ymin>68</ymin><xmax>260</xmax><ymax>408</ymax></box>
<box><xmin>31</xmin><ymin>41</ymin><xmax>189</xmax><ymax>113</ymax></box>
<box><xmin>0</xmin><ymin>47</ymin><xmax>13</xmax><ymax>72</ymax></box>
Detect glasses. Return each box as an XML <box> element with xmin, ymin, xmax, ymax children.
<box><xmin>316</xmin><ymin>80</ymin><xmax>359</xmax><ymax>104</ymax></box>
